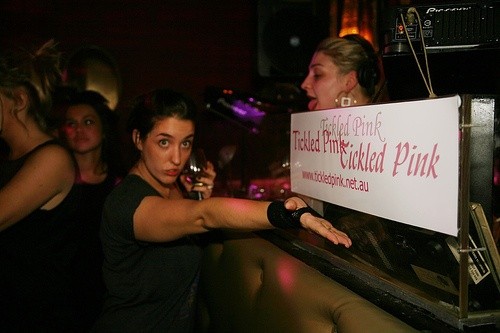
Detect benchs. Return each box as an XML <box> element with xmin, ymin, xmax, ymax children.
<box><xmin>188</xmin><ymin>225</ymin><xmax>448</xmax><ymax>333</ymax></box>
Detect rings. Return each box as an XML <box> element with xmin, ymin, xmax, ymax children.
<box><xmin>208</xmin><ymin>185</ymin><xmax>213</xmax><ymax>189</ymax></box>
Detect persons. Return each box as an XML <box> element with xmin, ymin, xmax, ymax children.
<box><xmin>0</xmin><ymin>38</ymin><xmax>120</xmax><ymax>333</ymax></box>
<box><xmin>201</xmin><ymin>82</ymin><xmax>309</xmax><ymax>183</ymax></box>
<box><xmin>302</xmin><ymin>34</ymin><xmax>424</xmax><ymax>265</ymax></box>
<box><xmin>89</xmin><ymin>88</ymin><xmax>353</xmax><ymax>333</ymax></box>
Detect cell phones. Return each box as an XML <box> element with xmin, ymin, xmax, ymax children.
<box><xmin>184</xmin><ymin>154</ymin><xmax>197</xmax><ymax>178</ymax></box>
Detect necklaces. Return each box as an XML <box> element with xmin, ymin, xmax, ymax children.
<box><xmin>136</xmin><ymin>163</ymin><xmax>144</xmax><ymax>178</ymax></box>
